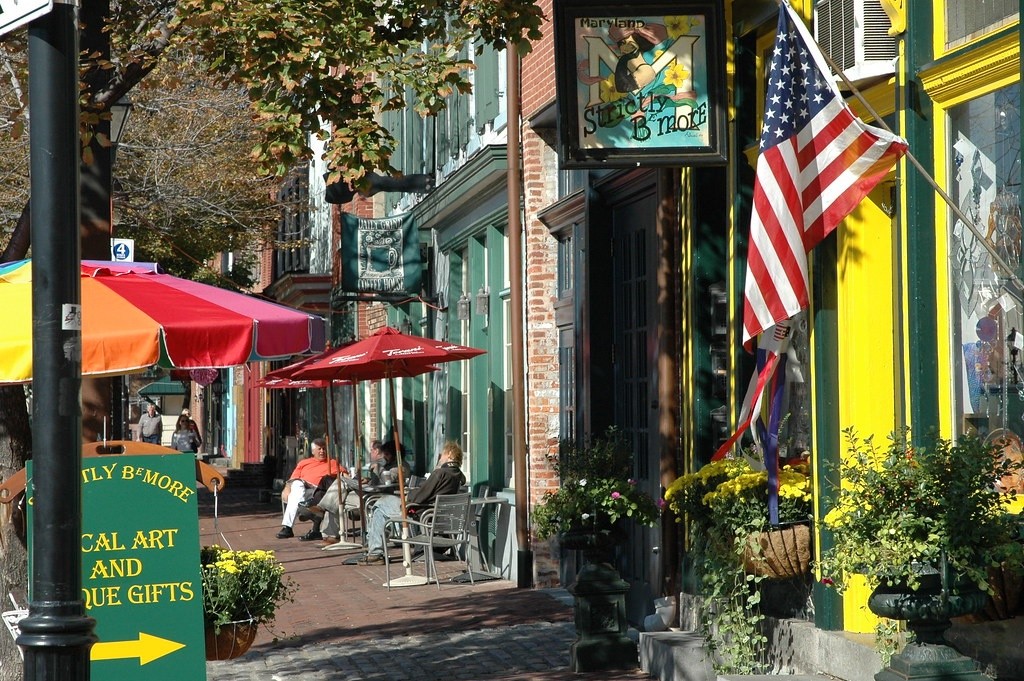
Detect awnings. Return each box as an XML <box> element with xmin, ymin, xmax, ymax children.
<box><xmin>138</xmin><ymin>377</ymin><xmax>186</xmax><ymax>396</ymax></box>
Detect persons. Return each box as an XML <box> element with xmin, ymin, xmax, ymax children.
<box><xmin>357</xmin><ymin>443</ymin><xmax>466</xmax><ymax>566</ymax></box>
<box><xmin>296</xmin><ymin>441</ymin><xmax>387</xmax><ymax>540</ymax></box>
<box><xmin>171</xmin><ymin>415</ymin><xmax>200</xmax><ymax>452</ymax></box>
<box><xmin>182</xmin><ymin>408</ymin><xmax>202</xmax><ymax>452</ymax></box>
<box><xmin>137</xmin><ymin>403</ymin><xmax>163</xmax><ymax>444</ymax></box>
<box><xmin>276</xmin><ymin>438</ymin><xmax>349</xmax><ymax>539</ymax></box>
<box><xmin>301</xmin><ymin>441</ymin><xmax>409</xmax><ymax>547</ymax></box>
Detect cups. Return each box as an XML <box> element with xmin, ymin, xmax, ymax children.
<box><xmin>383</xmin><ymin>471</ymin><xmax>392</xmax><ymax>485</ymax></box>
<box><xmin>188</xmin><ymin>433</ymin><xmax>194</xmax><ymax>443</ymax></box>
<box><xmin>350</xmin><ymin>467</ymin><xmax>357</xmax><ymax>479</ymax></box>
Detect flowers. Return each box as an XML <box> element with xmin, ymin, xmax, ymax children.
<box><xmin>661</xmin><ymin>455</ymin><xmax>818</xmax><ymax>533</ymax></box>
<box><xmin>197</xmin><ymin>543</ymin><xmax>300</xmax><ymax>644</ymax></box>
<box><xmin>531</xmin><ymin>424</ymin><xmax>657</xmax><ymax>545</ymax></box>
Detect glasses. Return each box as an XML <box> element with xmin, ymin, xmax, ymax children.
<box><xmin>184</xmin><ymin>413</ymin><xmax>190</xmax><ymax>415</ymax></box>
<box><xmin>181</xmin><ymin>420</ymin><xmax>189</xmax><ymax>422</ymax></box>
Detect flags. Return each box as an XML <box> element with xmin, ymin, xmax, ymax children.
<box><xmin>742</xmin><ymin>0</ymin><xmax>906</xmax><ymax>359</ymax></box>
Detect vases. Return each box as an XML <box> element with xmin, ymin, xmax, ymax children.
<box><xmin>205</xmin><ymin>621</ymin><xmax>258</xmax><ymax>661</ymax></box>
<box><xmin>714</xmin><ymin>524</ymin><xmax>811</xmax><ymax>582</ymax></box>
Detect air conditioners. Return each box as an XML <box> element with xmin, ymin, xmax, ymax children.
<box><xmin>813</xmin><ymin>0</ymin><xmax>899</xmax><ymax>92</ymax></box>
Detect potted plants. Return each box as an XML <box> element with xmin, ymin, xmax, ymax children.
<box><xmin>809</xmin><ymin>420</ymin><xmax>1024</xmax><ymax>681</ymax></box>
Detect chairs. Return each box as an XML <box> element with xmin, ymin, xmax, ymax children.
<box><xmin>382</xmin><ymin>492</ymin><xmax>475</xmax><ymax>592</ymax></box>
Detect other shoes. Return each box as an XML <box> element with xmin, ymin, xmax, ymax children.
<box><xmin>315</xmin><ymin>536</ymin><xmax>339</xmax><ymax>547</ymax></box>
<box><xmin>297</xmin><ymin>498</ymin><xmax>314</xmax><ymax>508</ymax></box>
<box><xmin>275</xmin><ymin>526</ymin><xmax>294</xmax><ymax>539</ymax></box>
<box><xmin>299</xmin><ymin>512</ymin><xmax>310</xmax><ymax>522</ymax></box>
<box><xmin>300</xmin><ymin>506</ymin><xmax>325</xmax><ymax>523</ymax></box>
<box><xmin>298</xmin><ymin>529</ymin><xmax>322</xmax><ymax>541</ymax></box>
<box><xmin>359</xmin><ymin>555</ymin><xmax>385</xmax><ymax>565</ymax></box>
<box><xmin>361</xmin><ymin>545</ymin><xmax>369</xmax><ymax>552</ymax></box>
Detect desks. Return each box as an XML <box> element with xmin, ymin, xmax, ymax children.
<box><xmin>431</xmin><ymin>496</ymin><xmax>508</xmax><ymax>583</ymax></box>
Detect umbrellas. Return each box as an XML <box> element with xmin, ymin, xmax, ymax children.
<box><xmin>0</xmin><ymin>258</ymin><xmax>326</xmax><ymax>383</ymax></box>
<box><xmin>253</xmin><ymin>334</ymin><xmax>441</xmax><ymax>551</ymax></box>
<box><xmin>289</xmin><ymin>326</ymin><xmax>486</xmax><ymax>588</ymax></box>
